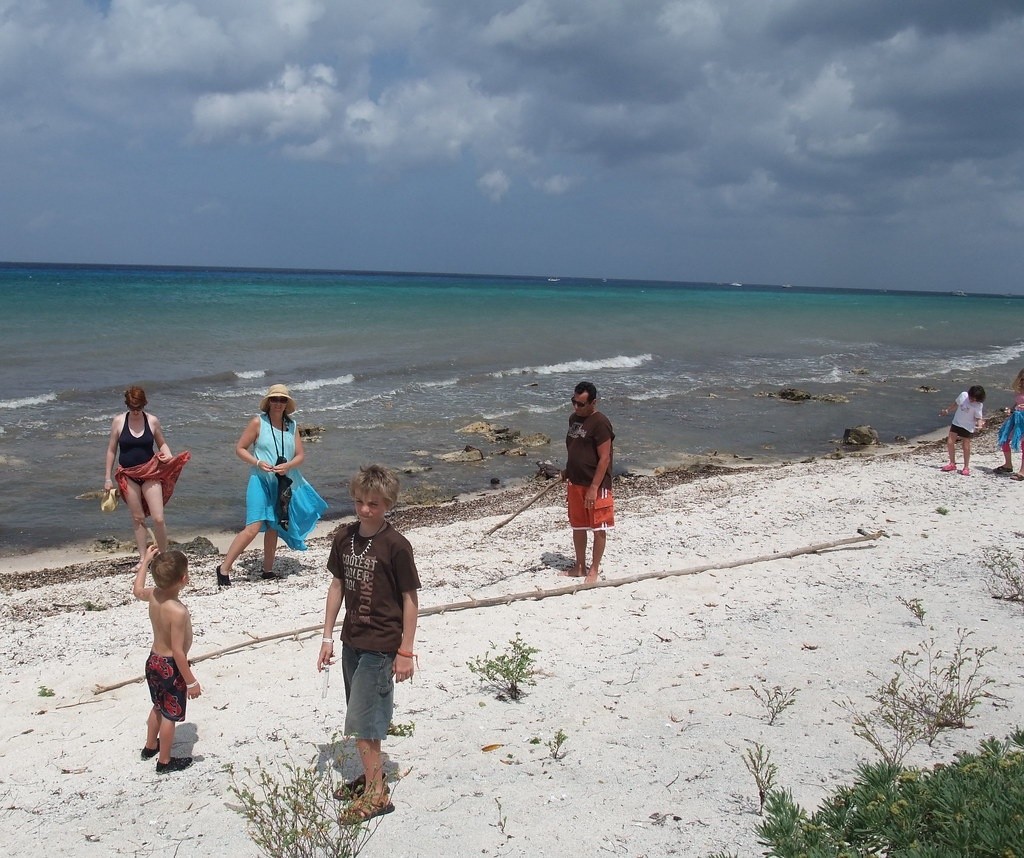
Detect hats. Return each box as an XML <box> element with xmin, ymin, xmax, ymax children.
<box><xmin>259</xmin><ymin>384</ymin><xmax>296</xmax><ymax>414</ymax></box>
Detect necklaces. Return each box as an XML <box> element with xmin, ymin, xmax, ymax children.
<box><xmin>351</xmin><ymin>520</ymin><xmax>385</xmax><ymax>560</ymax></box>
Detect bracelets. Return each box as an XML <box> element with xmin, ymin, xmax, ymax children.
<box><xmin>395</xmin><ymin>648</ymin><xmax>415</xmax><ymax>659</ymax></box>
<box><xmin>321</xmin><ymin>636</ymin><xmax>334</xmax><ymax>644</ymax></box>
<box><xmin>184</xmin><ymin>679</ymin><xmax>199</xmax><ymax>689</ymax></box>
<box><xmin>256</xmin><ymin>460</ymin><xmax>261</xmax><ymax>468</ymax></box>
<box><xmin>105</xmin><ymin>479</ymin><xmax>112</xmax><ymax>483</ymax></box>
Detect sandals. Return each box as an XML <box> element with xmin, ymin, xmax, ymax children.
<box><xmin>337</xmin><ymin>795</ymin><xmax>395</xmax><ymax>825</ymax></box>
<box><xmin>334</xmin><ymin>773</ymin><xmax>390</xmax><ymax>800</ymax></box>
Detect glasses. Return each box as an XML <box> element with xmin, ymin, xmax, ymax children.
<box><xmin>571</xmin><ymin>395</ymin><xmax>592</xmax><ymax>408</ymax></box>
<box><xmin>127</xmin><ymin>404</ymin><xmax>145</xmax><ymax>411</ymax></box>
<box><xmin>269</xmin><ymin>396</ymin><xmax>288</xmax><ymax>404</ymax></box>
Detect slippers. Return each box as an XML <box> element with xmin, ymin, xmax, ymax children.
<box><xmin>1010</xmin><ymin>473</ymin><xmax>1024</xmax><ymax>481</ymax></box>
<box><xmin>993</xmin><ymin>466</ymin><xmax>1013</xmax><ymax>472</ymax></box>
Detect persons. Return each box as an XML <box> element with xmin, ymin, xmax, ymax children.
<box><xmin>316</xmin><ymin>466</ymin><xmax>420</xmax><ymax>825</ymax></box>
<box><xmin>557</xmin><ymin>382</ymin><xmax>616</xmax><ymax>584</ymax></box>
<box><xmin>215</xmin><ymin>384</ymin><xmax>305</xmax><ymax>587</ymax></box>
<box><xmin>104</xmin><ymin>386</ymin><xmax>174</xmax><ymax>574</ymax></box>
<box><xmin>937</xmin><ymin>385</ymin><xmax>986</xmax><ymax>475</ymax></box>
<box><xmin>993</xmin><ymin>368</ymin><xmax>1024</xmax><ymax>480</ymax></box>
<box><xmin>135</xmin><ymin>544</ymin><xmax>202</xmax><ymax>776</ymax></box>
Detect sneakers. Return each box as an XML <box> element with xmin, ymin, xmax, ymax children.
<box><xmin>141</xmin><ymin>738</ymin><xmax>160</xmax><ymax>761</ymax></box>
<box><xmin>156</xmin><ymin>757</ymin><xmax>194</xmax><ymax>775</ymax></box>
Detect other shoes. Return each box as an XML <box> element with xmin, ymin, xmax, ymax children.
<box><xmin>130</xmin><ymin>561</ymin><xmax>143</xmax><ymax>573</ymax></box>
<box><xmin>217</xmin><ymin>566</ymin><xmax>231</xmax><ymax>590</ymax></box>
<box><xmin>942</xmin><ymin>463</ymin><xmax>957</xmax><ymax>470</ymax></box>
<box><xmin>961</xmin><ymin>467</ymin><xmax>970</xmax><ymax>475</ymax></box>
<box><xmin>261</xmin><ymin>571</ymin><xmax>285</xmax><ymax>581</ymax></box>
